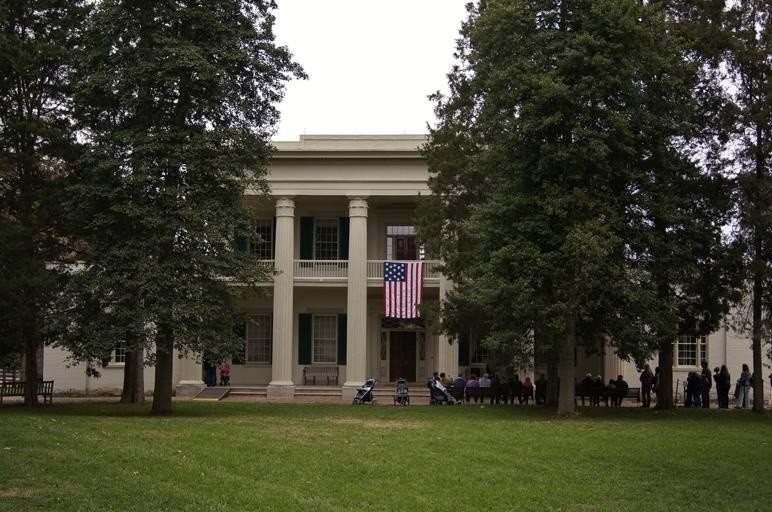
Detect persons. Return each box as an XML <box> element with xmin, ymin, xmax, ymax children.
<box><xmin>639</xmin><ymin>364</ymin><xmax>662</xmax><ymax>407</ymax></box>
<box><xmin>432</xmin><ymin>372</ymin><xmax>628</xmax><ymax>407</ymax></box>
<box><xmin>207</xmin><ymin>363</ymin><xmax>230</xmax><ymax>386</ymax></box>
<box><xmin>685</xmin><ymin>362</ymin><xmax>753</xmax><ymax>409</ymax></box>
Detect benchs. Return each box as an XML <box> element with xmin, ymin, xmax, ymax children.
<box><xmin>303</xmin><ymin>366</ymin><xmax>339</xmax><ymax>385</ymax></box>
<box><xmin>0</xmin><ymin>380</ymin><xmax>54</xmax><ymax>405</ymax></box>
<box><xmin>430</xmin><ymin>386</ymin><xmax>641</xmax><ymax>407</ymax></box>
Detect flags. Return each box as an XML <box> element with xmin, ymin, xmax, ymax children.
<box><xmin>384</xmin><ymin>260</ymin><xmax>423</xmax><ymax>319</ymax></box>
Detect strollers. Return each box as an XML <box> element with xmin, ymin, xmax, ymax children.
<box><xmin>392</xmin><ymin>376</ymin><xmax>413</xmax><ymax>406</ymax></box>
<box><xmin>426</xmin><ymin>377</ymin><xmax>457</xmax><ymax>405</ymax></box>
<box><xmin>352</xmin><ymin>376</ymin><xmax>380</xmax><ymax>404</ymax></box>
